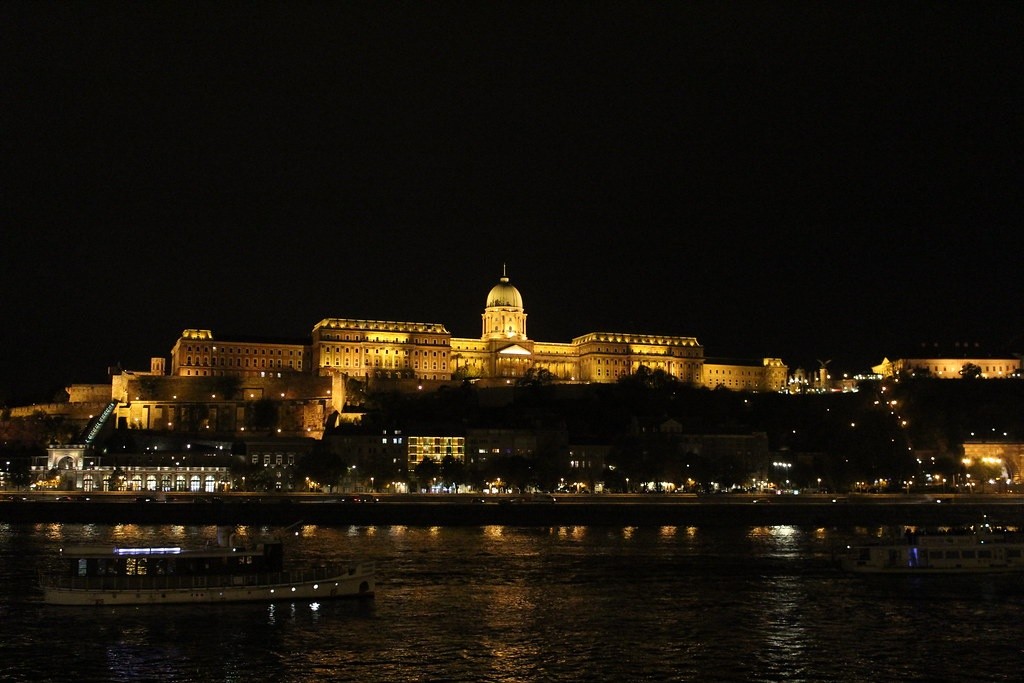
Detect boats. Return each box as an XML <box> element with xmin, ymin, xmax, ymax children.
<box><xmin>42</xmin><ymin>544</ymin><xmax>378</xmax><ymax>606</ymax></box>
<box><xmin>836</xmin><ymin>529</ymin><xmax>1024</xmax><ymax>571</ymax></box>
